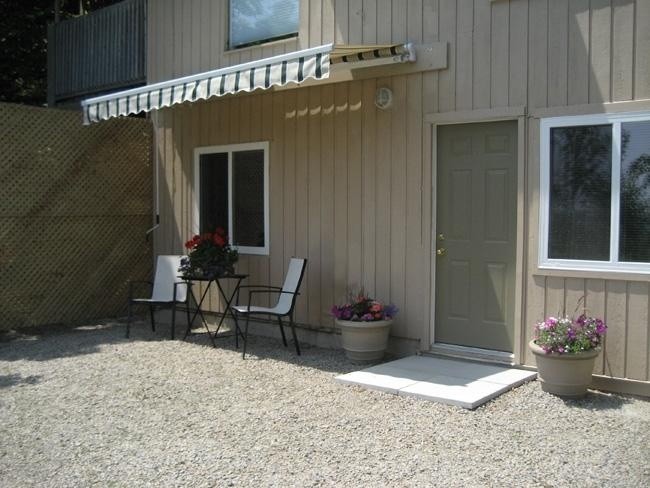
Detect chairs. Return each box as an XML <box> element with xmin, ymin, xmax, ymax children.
<box><xmin>125</xmin><ymin>253</ymin><xmax>192</xmax><ymax>341</ymax></box>
<box><xmin>230</xmin><ymin>256</ymin><xmax>308</xmax><ymax>360</ymax></box>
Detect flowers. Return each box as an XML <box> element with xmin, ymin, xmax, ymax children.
<box><xmin>534</xmin><ymin>309</ymin><xmax>608</xmax><ymax>357</ymax></box>
<box><xmin>330</xmin><ymin>292</ymin><xmax>399</xmax><ymax>321</ymax></box>
<box><xmin>178</xmin><ymin>226</ymin><xmax>239</xmax><ymax>277</ymax></box>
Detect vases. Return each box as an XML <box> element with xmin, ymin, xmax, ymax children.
<box><xmin>528</xmin><ymin>338</ymin><xmax>600</xmax><ymax>401</ymax></box>
<box><xmin>335</xmin><ymin>318</ymin><xmax>393</xmax><ymax>361</ymax></box>
<box><xmin>203</xmin><ymin>264</ymin><xmax>226</xmax><ymax>277</ymax></box>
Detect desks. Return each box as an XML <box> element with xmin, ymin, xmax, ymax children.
<box><xmin>176</xmin><ymin>273</ymin><xmax>246</xmax><ymax>348</ymax></box>
